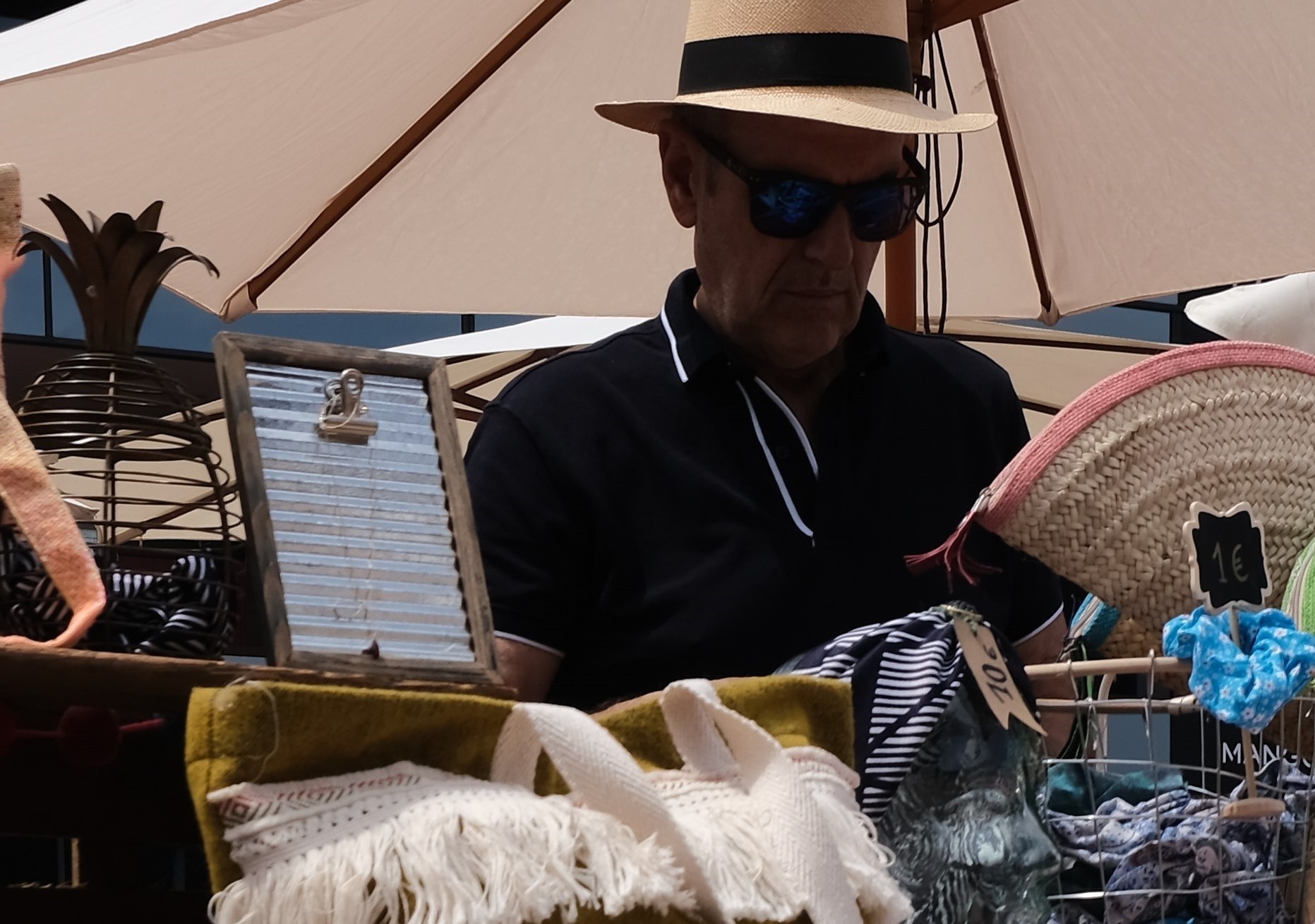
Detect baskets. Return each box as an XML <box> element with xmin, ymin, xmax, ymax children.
<box><xmin>1038</xmin><ymin>646</ymin><xmax>1313</xmax><ymax>924</ymax></box>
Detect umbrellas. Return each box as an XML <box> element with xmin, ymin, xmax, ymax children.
<box><xmin>0</xmin><ymin>0</ymin><xmax>1315</xmax><ymax>330</ymax></box>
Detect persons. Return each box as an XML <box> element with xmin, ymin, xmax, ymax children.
<box><xmin>463</xmin><ymin>0</ymin><xmax>1088</xmax><ymax>771</ymax></box>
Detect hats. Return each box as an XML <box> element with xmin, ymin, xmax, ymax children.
<box><xmin>593</xmin><ymin>0</ymin><xmax>1000</xmax><ymax>139</ymax></box>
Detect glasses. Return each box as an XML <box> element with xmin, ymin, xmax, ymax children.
<box><xmin>686</xmin><ymin>118</ymin><xmax>931</xmax><ymax>242</ymax></box>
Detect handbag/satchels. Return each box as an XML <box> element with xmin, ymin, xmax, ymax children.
<box><xmin>768</xmin><ymin>599</ymin><xmax>1029</xmax><ymax>924</ymax></box>
<box><xmin>183</xmin><ymin>671</ymin><xmax>916</xmax><ymax>923</ymax></box>
<box><xmin>900</xmin><ymin>340</ymin><xmax>1315</xmax><ymax>697</ymax></box>
